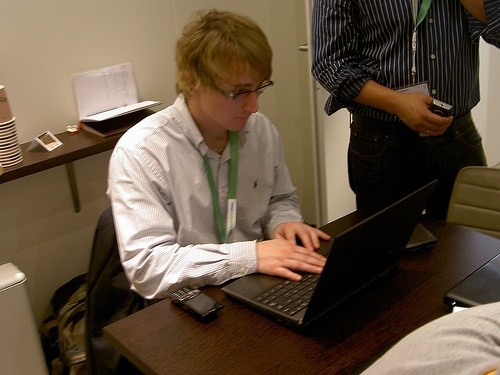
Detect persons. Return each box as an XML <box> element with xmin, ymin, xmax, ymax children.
<box><xmin>106</xmin><ymin>9</ymin><xmax>332</xmax><ymax>309</ymax></box>
<box><xmin>309</xmin><ymin>0</ymin><xmax>500</xmax><ymax>212</ymax></box>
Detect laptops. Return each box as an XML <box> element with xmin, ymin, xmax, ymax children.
<box><xmin>221</xmin><ymin>178</ymin><xmax>441</xmax><ymax>330</ymax></box>
<box><xmin>443</xmin><ymin>253</ymin><xmax>500</xmax><ymax>309</ymax></box>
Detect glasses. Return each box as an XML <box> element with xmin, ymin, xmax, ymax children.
<box><xmin>212</xmin><ymin>79</ymin><xmax>273</xmax><ymax>101</ymax></box>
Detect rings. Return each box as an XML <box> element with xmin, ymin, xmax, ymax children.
<box><xmin>427</xmin><ymin>129</ymin><xmax>431</xmax><ymax>135</ymax></box>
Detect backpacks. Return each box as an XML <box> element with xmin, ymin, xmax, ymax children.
<box><xmin>41</xmin><ymin>272</ymin><xmax>89</xmax><ymax>375</ymax></box>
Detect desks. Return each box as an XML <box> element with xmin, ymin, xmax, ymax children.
<box><xmin>102</xmin><ymin>180</ymin><xmax>500</xmax><ymax>375</ymax></box>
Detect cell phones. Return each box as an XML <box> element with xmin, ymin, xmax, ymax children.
<box><xmin>429</xmin><ymin>100</ymin><xmax>453</xmax><ymax>117</ymax></box>
<box><xmin>171</xmin><ymin>285</ymin><xmax>224</xmax><ymax>320</ymax></box>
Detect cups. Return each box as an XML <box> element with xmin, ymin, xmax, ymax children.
<box><xmin>0</xmin><ymin>84</ymin><xmax>24</xmax><ymax>168</ymax></box>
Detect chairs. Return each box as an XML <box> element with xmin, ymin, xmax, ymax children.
<box><xmin>446</xmin><ymin>166</ymin><xmax>500</xmax><ymax>239</ymax></box>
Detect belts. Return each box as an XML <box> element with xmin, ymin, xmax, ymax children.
<box><xmin>353</xmin><ymin>112</ymin><xmax>472</xmax><ymax>137</ymax></box>
